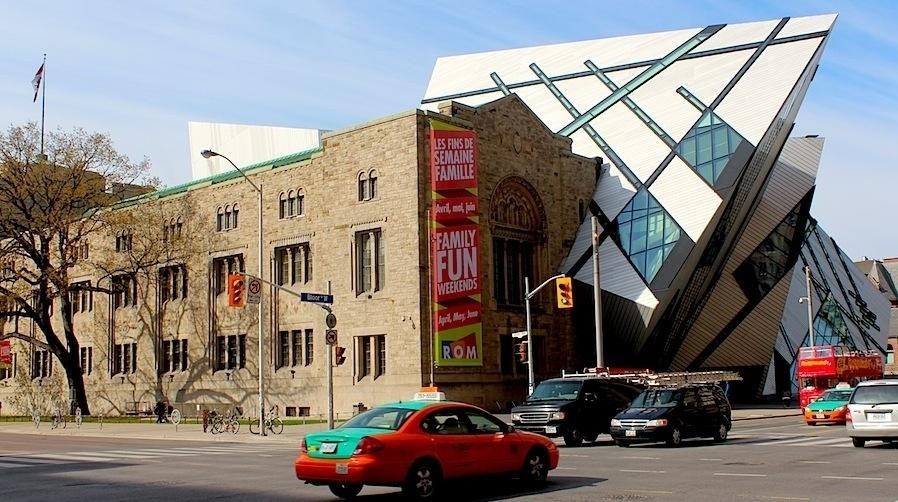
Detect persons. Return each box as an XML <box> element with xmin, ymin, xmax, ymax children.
<box><xmin>155</xmin><ymin>382</ymin><xmax>166</xmax><ymax>423</ymax></box>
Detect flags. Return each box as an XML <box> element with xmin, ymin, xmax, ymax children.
<box><xmin>30</xmin><ymin>63</ymin><xmax>44</xmax><ymax>102</ymax></box>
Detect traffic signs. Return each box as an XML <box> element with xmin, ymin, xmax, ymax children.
<box><xmin>300</xmin><ymin>293</ymin><xmax>334</xmax><ymax>303</ymax></box>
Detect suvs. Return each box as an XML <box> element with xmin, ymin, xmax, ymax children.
<box><xmin>510</xmin><ymin>378</ymin><xmax>732</xmax><ymax>448</ymax></box>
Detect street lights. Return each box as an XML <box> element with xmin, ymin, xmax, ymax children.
<box><xmin>798</xmin><ymin>266</ymin><xmax>815</xmax><ymax>347</ymax></box>
<box><xmin>201</xmin><ymin>150</ymin><xmax>264</xmax><ymax>435</ymax></box>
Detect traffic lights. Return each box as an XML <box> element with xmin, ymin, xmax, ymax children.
<box><xmin>556</xmin><ymin>277</ymin><xmax>574</xmax><ymax>308</ymax></box>
<box><xmin>335</xmin><ymin>346</ymin><xmax>346</xmax><ymax>365</ymax></box>
<box><xmin>228</xmin><ymin>274</ymin><xmax>245</xmax><ymax>306</ymax></box>
<box><xmin>513</xmin><ymin>342</ymin><xmax>527</xmax><ymax>361</ymax></box>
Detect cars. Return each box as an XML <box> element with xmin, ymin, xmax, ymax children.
<box><xmin>804</xmin><ymin>387</ymin><xmax>855</xmax><ymax>426</ymax></box>
<box><xmin>296</xmin><ymin>398</ymin><xmax>559</xmax><ymax>500</ymax></box>
<box><xmin>846</xmin><ymin>378</ymin><xmax>898</xmax><ymax>447</ymax></box>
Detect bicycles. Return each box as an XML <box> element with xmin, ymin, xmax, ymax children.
<box><xmin>205</xmin><ymin>408</ymin><xmax>283</xmax><ymax>435</ymax></box>
<box><xmin>51</xmin><ymin>407</ymin><xmax>67</xmax><ymax>429</ymax></box>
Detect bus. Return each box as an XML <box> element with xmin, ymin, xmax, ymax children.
<box><xmin>797</xmin><ymin>345</ymin><xmax>885</xmax><ymax>414</ymax></box>
